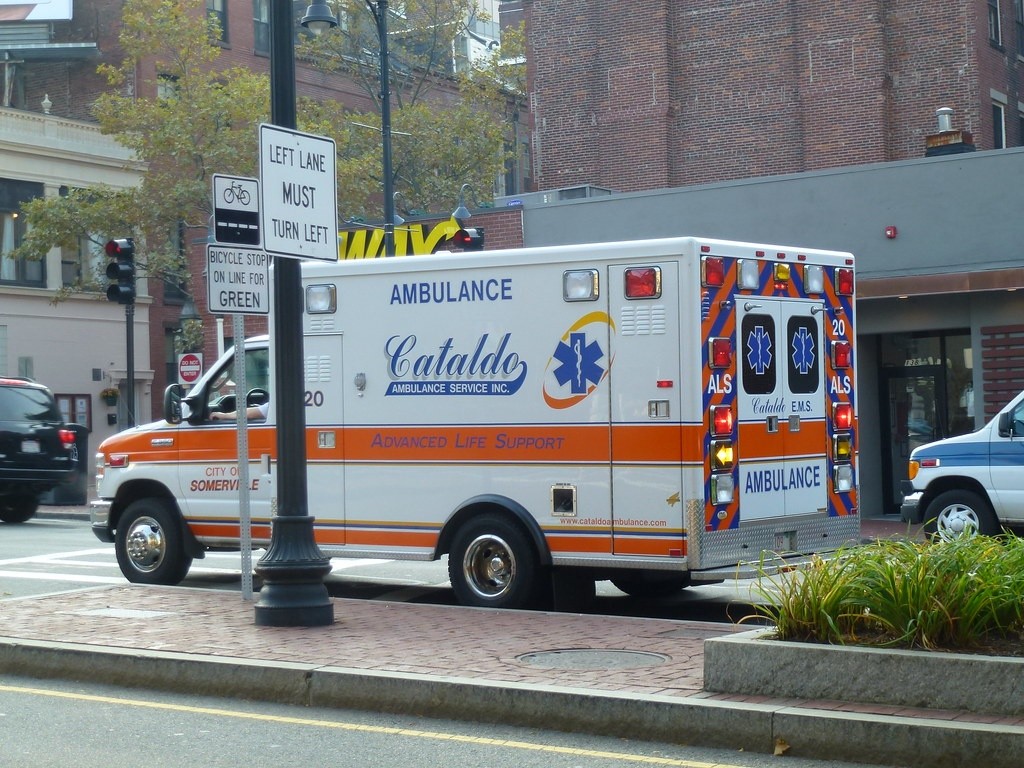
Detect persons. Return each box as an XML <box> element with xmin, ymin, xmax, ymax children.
<box><xmin>960</xmin><ymin>382</ymin><xmax>975</xmax><ymax>433</ymax></box>
<box><xmin>908</xmin><ymin>376</ymin><xmax>928</xmax><ymax>437</ymax></box>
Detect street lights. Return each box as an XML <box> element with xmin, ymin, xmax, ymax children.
<box><xmin>300</xmin><ymin>1</ymin><xmax>396</xmax><ymax>257</ymax></box>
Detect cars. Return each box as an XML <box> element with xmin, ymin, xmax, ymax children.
<box><xmin>902</xmin><ymin>392</ymin><xmax>1024</xmax><ymax>545</ymax></box>
<box><xmin>0</xmin><ymin>376</ymin><xmax>74</xmax><ymax>524</ymax></box>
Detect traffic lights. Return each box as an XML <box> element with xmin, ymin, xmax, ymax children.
<box><xmin>105</xmin><ymin>239</ymin><xmax>132</xmax><ymax>304</ymax></box>
<box><xmin>453</xmin><ymin>228</ymin><xmax>480</xmax><ymax>249</ymax></box>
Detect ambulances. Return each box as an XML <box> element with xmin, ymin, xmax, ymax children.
<box><xmin>85</xmin><ymin>237</ymin><xmax>861</xmax><ymax>608</ymax></box>
